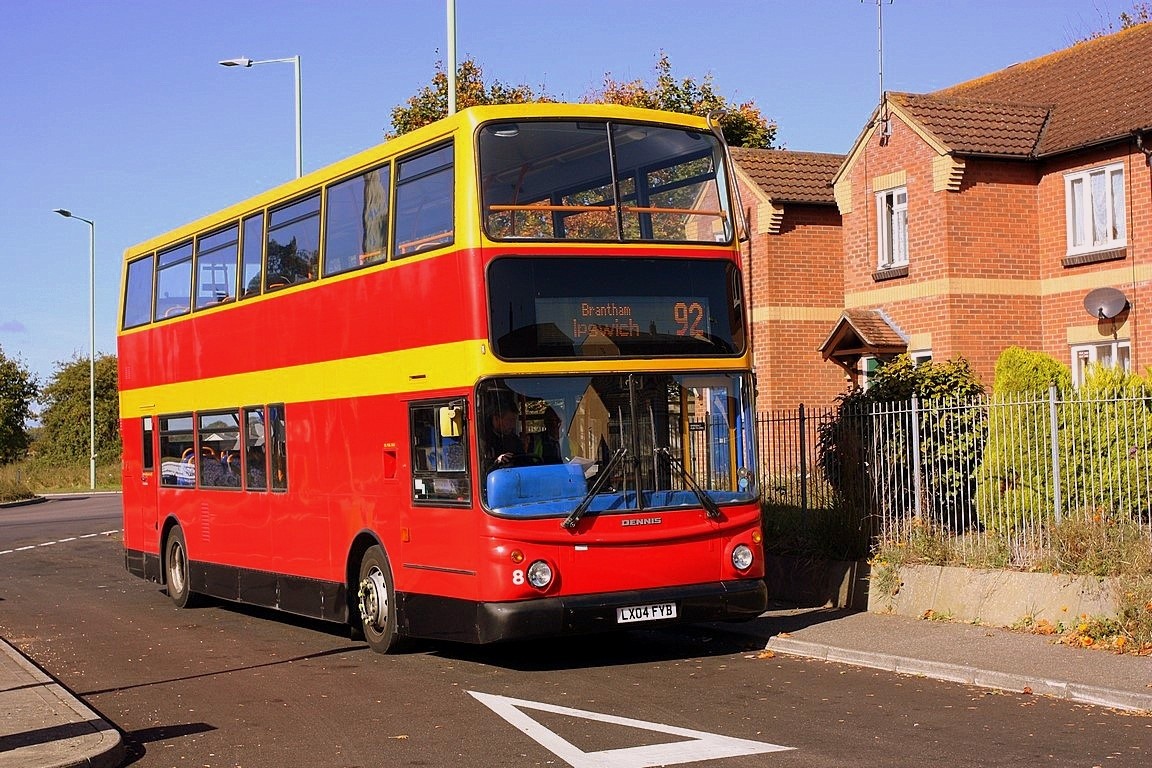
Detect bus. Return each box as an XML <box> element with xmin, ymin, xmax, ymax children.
<box><xmin>118</xmin><ymin>103</ymin><xmax>769</xmax><ymax>654</ymax></box>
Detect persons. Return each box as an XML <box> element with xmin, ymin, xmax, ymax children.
<box><xmin>481</xmin><ymin>397</ymin><xmax>524</xmax><ymax>471</ymax></box>
<box><xmin>532</xmin><ymin>404</ymin><xmax>577</xmax><ymax>466</ymax></box>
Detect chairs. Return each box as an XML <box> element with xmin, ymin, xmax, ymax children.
<box><xmin>164</xmin><ymin>281</ymin><xmax>284</xmax><ymax>318</ymax></box>
<box><xmin>177</xmin><ymin>447</ymin><xmax>261</xmax><ymax>485</ymax></box>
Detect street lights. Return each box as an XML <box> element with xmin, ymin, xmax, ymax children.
<box><xmin>52</xmin><ymin>208</ymin><xmax>96</xmax><ymax>489</ymax></box>
<box><xmin>219</xmin><ymin>55</ymin><xmax>303</xmax><ymax>181</ymax></box>
